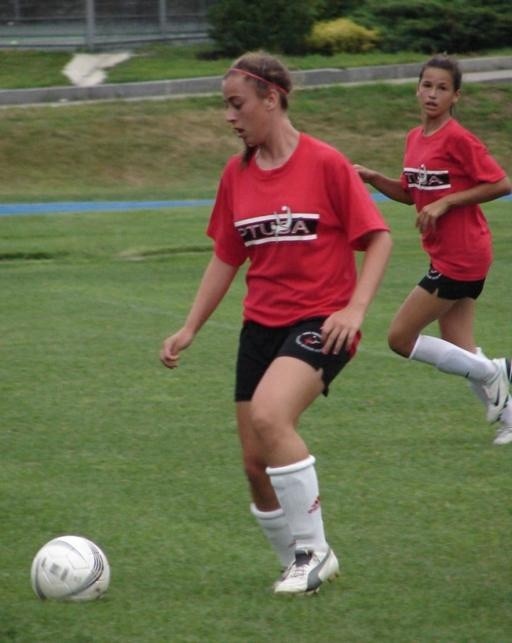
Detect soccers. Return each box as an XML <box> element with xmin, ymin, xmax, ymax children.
<box><xmin>31</xmin><ymin>535</ymin><xmax>110</xmax><ymax>602</ymax></box>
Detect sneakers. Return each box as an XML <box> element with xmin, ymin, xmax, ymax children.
<box><xmin>272</xmin><ymin>543</ymin><xmax>339</xmax><ymax>596</ymax></box>
<box><xmin>480</xmin><ymin>357</ymin><xmax>512</xmax><ymax>445</ymax></box>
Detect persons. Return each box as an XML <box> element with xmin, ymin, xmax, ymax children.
<box><xmin>351</xmin><ymin>51</ymin><xmax>511</xmax><ymax>445</ymax></box>
<box><xmin>158</xmin><ymin>50</ymin><xmax>395</xmax><ymax>597</ymax></box>
